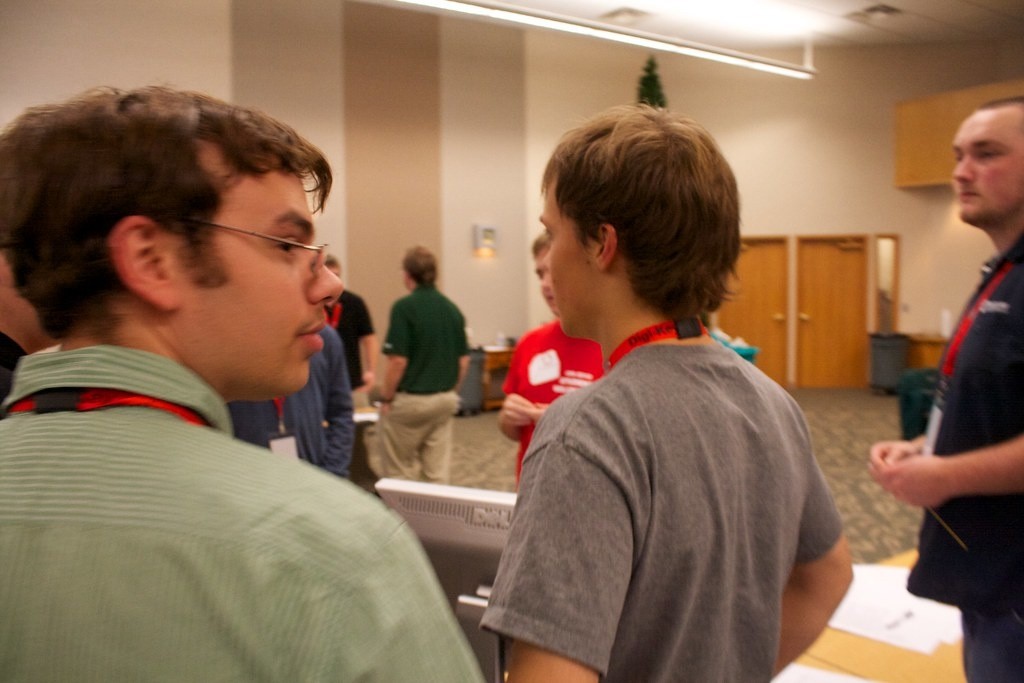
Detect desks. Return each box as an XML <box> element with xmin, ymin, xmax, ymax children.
<box><xmin>771</xmin><ymin>550</ymin><xmax>972</xmax><ymax>683</ymax></box>
<box><xmin>478</xmin><ymin>349</ymin><xmax>516</xmax><ymax>411</ymax></box>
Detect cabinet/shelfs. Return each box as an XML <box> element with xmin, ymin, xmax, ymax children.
<box><xmin>894</xmin><ymin>78</ymin><xmax>1024</xmax><ymax>188</ymax></box>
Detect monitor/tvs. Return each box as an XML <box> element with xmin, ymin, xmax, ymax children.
<box><xmin>370</xmin><ymin>477</ymin><xmax>519</xmax><ymax>683</ymax></box>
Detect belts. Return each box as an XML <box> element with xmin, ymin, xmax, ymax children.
<box><xmin>396</xmin><ymin>387</ymin><xmax>456</xmax><ymax>394</ymax></box>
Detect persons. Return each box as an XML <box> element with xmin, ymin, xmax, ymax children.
<box><xmin>368</xmin><ymin>247</ymin><xmax>472</xmax><ymax>486</ymax></box>
<box><xmin>498</xmin><ymin>233</ymin><xmax>607</xmax><ymax>489</ymax></box>
<box><xmin>0</xmin><ymin>252</ymin><xmax>61</xmax><ymax>404</ymax></box>
<box><xmin>0</xmin><ymin>88</ymin><xmax>484</xmax><ymax>682</ymax></box>
<box><xmin>323</xmin><ymin>256</ymin><xmax>379</xmax><ymax>491</ymax></box>
<box><xmin>867</xmin><ymin>94</ymin><xmax>1024</xmax><ymax>683</ymax></box>
<box><xmin>698</xmin><ymin>311</ymin><xmax>758</xmax><ymax>362</ymax></box>
<box><xmin>478</xmin><ymin>107</ymin><xmax>854</xmax><ymax>683</ymax></box>
<box><xmin>227</xmin><ymin>321</ymin><xmax>353</xmax><ymax>481</ymax></box>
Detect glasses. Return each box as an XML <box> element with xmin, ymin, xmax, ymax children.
<box><xmin>154</xmin><ymin>215</ymin><xmax>329</xmax><ymax>272</ymax></box>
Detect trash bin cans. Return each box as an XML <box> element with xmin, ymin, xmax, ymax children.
<box><xmin>455</xmin><ymin>348</ymin><xmax>484</xmax><ymax>416</ymax></box>
<box><xmin>869</xmin><ymin>332</ymin><xmax>911</xmax><ymax>390</ymax></box>
